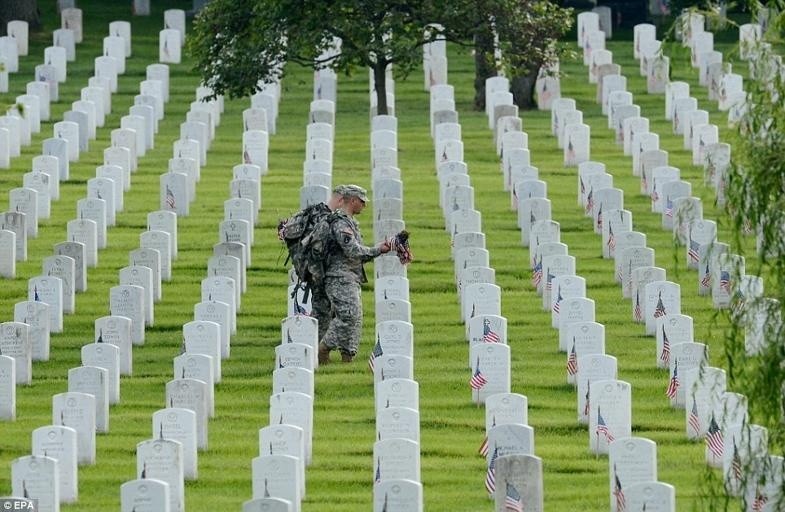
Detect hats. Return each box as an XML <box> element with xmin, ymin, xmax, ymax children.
<box><xmin>345</xmin><ymin>184</ymin><xmax>370</xmax><ymax>202</ymax></box>
<box><xmin>333</xmin><ymin>185</ymin><xmax>346</xmax><ymax>197</ymax></box>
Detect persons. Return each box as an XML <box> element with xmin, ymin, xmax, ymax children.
<box><xmin>318</xmin><ymin>183</ymin><xmax>391</xmax><ymax>365</ymax></box>
<box><xmin>310</xmin><ymin>183</ymin><xmax>346</xmax><ymax>342</ymax></box>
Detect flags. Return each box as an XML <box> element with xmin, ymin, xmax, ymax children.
<box><xmin>376</xmin><ymin>190</ymin><xmax>398</xmax><ymax>512</ymax></box>
<box><xmin>499</xmin><ymin>127</ymin><xmax>627</xmax><ymax>511</ymax></box>
<box><xmin>563</xmin><ymin>139</ymin><xmax>766</xmax><ymax>511</ymax></box>
<box><xmin>450</xmin><ymin>223</ymin><xmax>521</xmax><ymax>510</ymax></box>
<box><xmin>581</xmin><ymin>16</ymin><xmax>771</xmax><ymax>319</ymax></box>
<box><xmin>244</xmin><ymin>151</ymin><xmax>251</xmax><ymax>163</ymax></box>
<box><xmin>167</xmin><ymin>189</ymin><xmax>175</xmax><ymax>208</ymax></box>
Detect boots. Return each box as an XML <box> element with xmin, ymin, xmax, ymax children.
<box><xmin>341</xmin><ymin>353</ymin><xmax>354</xmax><ymax>363</ymax></box>
<box><xmin>318</xmin><ymin>339</ymin><xmax>331</xmax><ymax>364</ymax></box>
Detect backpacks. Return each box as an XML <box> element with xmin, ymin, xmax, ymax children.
<box><xmin>284</xmin><ymin>203</ymin><xmax>328</xmax><ymax>282</ymax></box>
<box><xmin>299</xmin><ymin>212</ymin><xmax>357</xmax><ymax>290</ymax></box>
